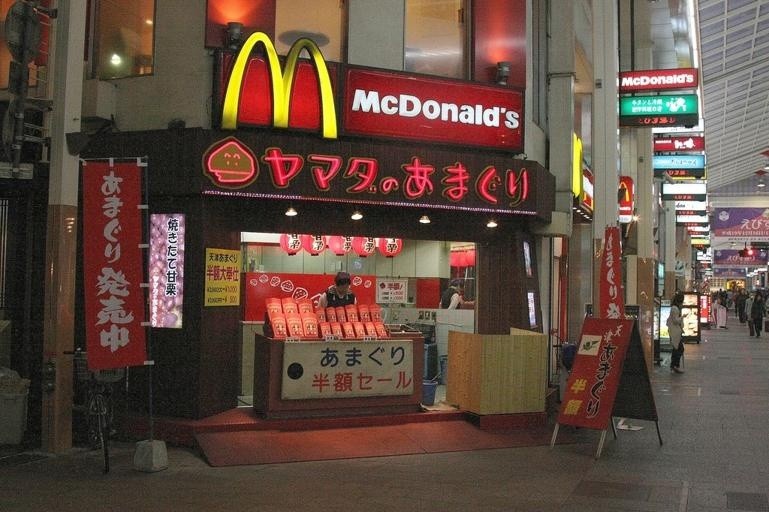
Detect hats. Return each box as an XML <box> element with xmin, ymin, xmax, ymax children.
<box><xmin>451</xmin><ymin>279</ymin><xmax>464</xmax><ymax>286</ymax></box>
<box><xmin>335</xmin><ymin>272</ymin><xmax>350</xmax><ymax>285</ymax></box>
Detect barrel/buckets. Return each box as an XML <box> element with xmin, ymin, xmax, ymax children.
<box><xmin>0</xmin><ymin>383</ymin><xmax>30</xmax><ymax>445</ymax></box>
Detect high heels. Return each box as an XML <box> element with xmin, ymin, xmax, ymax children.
<box><xmin>670</xmin><ymin>366</ymin><xmax>684</xmax><ymax>374</ymax></box>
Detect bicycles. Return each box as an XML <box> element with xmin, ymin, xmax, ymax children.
<box><xmin>63</xmin><ymin>348</ymin><xmax>131</xmax><ymax>472</ymax></box>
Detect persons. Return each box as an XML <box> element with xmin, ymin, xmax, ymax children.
<box><xmin>318</xmin><ymin>272</ymin><xmax>358</xmax><ymax>308</ymax></box>
<box><xmin>667</xmin><ymin>291</ymin><xmax>688</xmax><ymax>375</ymax></box>
<box><xmin>437</xmin><ymin>278</ymin><xmax>461</xmax><ymax>309</ymax></box>
<box><xmin>710</xmin><ymin>286</ymin><xmax>765</xmax><ymax>339</ymax></box>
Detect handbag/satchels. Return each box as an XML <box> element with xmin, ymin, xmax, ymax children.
<box><xmin>765</xmin><ymin>321</ymin><xmax>769</xmax><ymax>332</ymax></box>
<box><xmin>750</xmin><ymin>326</ymin><xmax>754</xmax><ymax>336</ymax></box>
